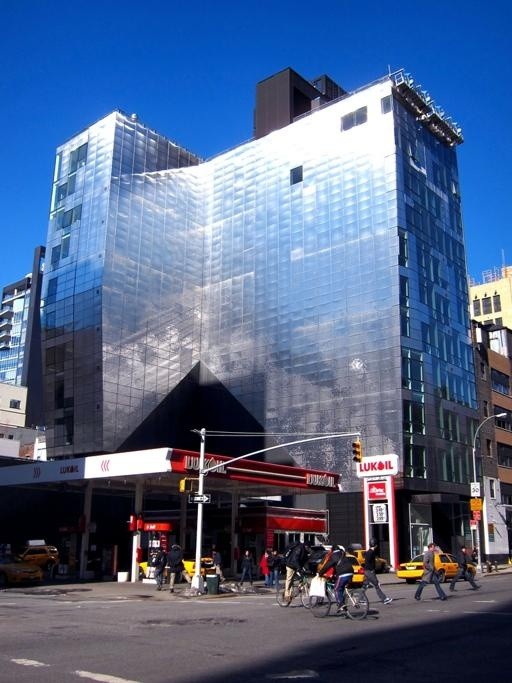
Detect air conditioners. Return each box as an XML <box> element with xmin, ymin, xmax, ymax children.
<box><xmin>475</xmin><ymin>328</ymin><xmax>487</xmax><ymax>344</ymax></box>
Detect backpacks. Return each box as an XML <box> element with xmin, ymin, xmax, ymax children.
<box><xmin>283</xmin><ymin>541</ymin><xmax>303</xmax><ymax>559</ymax></box>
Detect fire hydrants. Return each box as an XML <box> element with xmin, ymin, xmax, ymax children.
<box><xmin>486</xmin><ymin>560</ymin><xmax>492</xmax><ymax>573</ymax></box>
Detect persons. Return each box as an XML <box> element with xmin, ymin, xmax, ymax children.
<box><xmin>260</xmin><ymin>539</ymin><xmax>393</xmax><ymax>613</ymax></box>
<box><xmin>414</xmin><ymin>543</ymin><xmax>448</xmax><ymax>600</ymax></box>
<box><xmin>212</xmin><ymin>550</ymin><xmax>227</xmax><ymax>583</ymax></box>
<box><xmin>167</xmin><ymin>544</ymin><xmax>191</xmax><ymax>592</ymax></box>
<box><xmin>450</xmin><ymin>547</ymin><xmax>480</xmax><ymax>592</ymax></box>
<box><xmin>153</xmin><ymin>547</ymin><xmax>166</xmax><ymax>590</ymax></box>
<box><xmin>238</xmin><ymin>549</ymin><xmax>258</xmax><ymax>587</ymax></box>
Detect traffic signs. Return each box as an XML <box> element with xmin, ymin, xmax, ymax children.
<box><xmin>471</xmin><ymin>482</ymin><xmax>481</xmax><ymax>498</ymax></box>
<box><xmin>189</xmin><ymin>494</ymin><xmax>211</xmax><ymax>503</ymax></box>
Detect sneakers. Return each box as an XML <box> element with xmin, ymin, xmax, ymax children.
<box><xmin>383</xmin><ymin>597</ymin><xmax>392</xmax><ymax>605</ymax></box>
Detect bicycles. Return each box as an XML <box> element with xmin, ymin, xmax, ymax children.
<box><xmin>305</xmin><ymin>579</ymin><xmax>369</xmax><ymax>619</ymax></box>
<box><xmin>276</xmin><ymin>571</ymin><xmax>319</xmax><ymax>608</ymax></box>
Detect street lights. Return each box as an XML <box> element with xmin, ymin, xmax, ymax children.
<box><xmin>470</xmin><ymin>413</ymin><xmax>508</xmax><ymax>573</ymax></box>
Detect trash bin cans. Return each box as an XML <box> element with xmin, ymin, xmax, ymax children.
<box><xmin>206</xmin><ymin>574</ymin><xmax>220</xmax><ymax>595</ymax></box>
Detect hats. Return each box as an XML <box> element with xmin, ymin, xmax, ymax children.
<box><xmin>370</xmin><ymin>537</ymin><xmax>377</xmax><ymax>546</ymax></box>
<box><xmin>303</xmin><ymin>540</ymin><xmax>311</xmax><ymax>548</ymax></box>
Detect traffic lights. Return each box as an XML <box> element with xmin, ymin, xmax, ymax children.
<box><xmin>185</xmin><ymin>480</ymin><xmax>192</xmax><ymax>490</ymax></box>
<box><xmin>352</xmin><ymin>441</ymin><xmax>362</xmax><ymax>463</ymax></box>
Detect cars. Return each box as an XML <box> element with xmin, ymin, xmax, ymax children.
<box><xmin>308</xmin><ymin>550</ymin><xmax>331</xmax><ymax>572</ymax></box>
<box><xmin>15</xmin><ymin>540</ymin><xmax>59</xmax><ymax>567</ymax></box>
<box><xmin>140</xmin><ymin>556</ymin><xmax>205</xmax><ymax>581</ymax></box>
<box><xmin>348</xmin><ymin>543</ymin><xmax>391</xmax><ymax>572</ymax></box>
<box><xmin>396</xmin><ymin>546</ymin><xmax>476</xmax><ymax>584</ymax></box>
<box><xmin>1</xmin><ymin>552</ymin><xmax>44</xmax><ymax>588</ymax></box>
<box><xmin>189</xmin><ymin>555</ymin><xmax>216</xmax><ymax>574</ymax></box>
<box><xmin>320</xmin><ymin>552</ymin><xmax>367</xmax><ymax>585</ymax></box>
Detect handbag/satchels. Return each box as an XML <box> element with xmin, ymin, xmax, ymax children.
<box><xmin>308</xmin><ymin>572</ymin><xmax>326</xmax><ymax>597</ymax></box>
